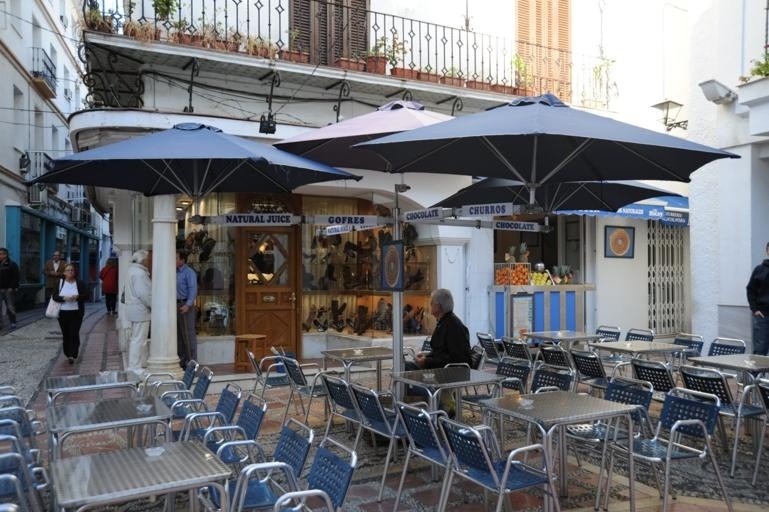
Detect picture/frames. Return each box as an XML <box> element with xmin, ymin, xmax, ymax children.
<box><xmin>379</xmin><ymin>240</ymin><xmax>405</xmax><ymax>291</ymax></box>
<box><xmin>603</xmin><ymin>223</ymin><xmax>635</xmax><ymax>259</ymax></box>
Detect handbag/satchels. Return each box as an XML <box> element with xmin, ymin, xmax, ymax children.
<box><xmin>44</xmin><ymin>295</ymin><xmax>60</xmax><ymax>319</ymax></box>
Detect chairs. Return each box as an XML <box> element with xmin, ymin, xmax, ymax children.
<box><xmin>1</xmin><ymin>383</ymin><xmax>52</xmax><ymax>512</ymax></box>
<box><xmin>130</xmin><ymin>326</ymin><xmax>769</xmax><ymax>511</ymax></box>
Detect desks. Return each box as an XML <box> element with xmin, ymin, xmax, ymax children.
<box><xmin>44</xmin><ymin>369</ymin><xmax>234</xmax><ymax>512</ymax></box>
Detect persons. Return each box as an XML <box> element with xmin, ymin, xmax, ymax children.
<box><xmin>745</xmin><ymin>240</ymin><xmax>769</xmax><ymax>356</ymax></box>
<box><xmin>0</xmin><ymin>248</ymin><xmax>153</xmax><ymax>375</ymax></box>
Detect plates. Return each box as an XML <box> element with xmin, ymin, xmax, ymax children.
<box><xmin>352</xmin><ymin>349</ymin><xmax>362</xmax><ymax>354</ymax></box>
<box><xmin>518</xmin><ymin>399</ymin><xmax>533</xmax><ymax>406</ymax></box>
<box><xmin>422</xmin><ymin>373</ymin><xmax>435</xmax><ymax>378</ymax></box>
<box><xmin>135</xmin><ymin>404</ymin><xmax>152</xmax><ymax>411</ymax></box>
<box><xmin>144</xmin><ymin>446</ymin><xmax>164</xmax><ymax>456</ymax></box>
<box><xmin>745</xmin><ymin>360</ymin><xmax>757</xmax><ymax>365</ymax></box>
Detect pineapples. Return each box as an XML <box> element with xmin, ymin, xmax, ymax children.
<box><xmin>518</xmin><ymin>254</ymin><xmax>527</xmax><ymax>262</ymax></box>
<box><xmin>555</xmin><ymin>274</ymin><xmax>574</xmax><ymax>284</ymax></box>
<box><xmin>507</xmin><ymin>256</ymin><xmax>516</xmax><ymax>263</ymax></box>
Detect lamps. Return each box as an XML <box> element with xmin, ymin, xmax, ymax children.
<box><xmin>651</xmin><ymin>99</ymin><xmax>690</xmax><ymax>134</ymax></box>
<box><xmin>259</xmin><ymin>58</ymin><xmax>281</xmax><ymax>136</ymax></box>
<box><xmin>697</xmin><ymin>77</ymin><xmax>740</xmax><ymax>107</ymax></box>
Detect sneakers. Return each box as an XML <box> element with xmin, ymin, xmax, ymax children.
<box><xmin>11</xmin><ymin>323</ymin><xmax>16</xmax><ymax>332</ymax></box>
<box><xmin>106</xmin><ymin>311</ymin><xmax>117</xmax><ymax>316</ymax></box>
<box><xmin>68</xmin><ymin>349</ymin><xmax>79</xmax><ymax>366</ymax></box>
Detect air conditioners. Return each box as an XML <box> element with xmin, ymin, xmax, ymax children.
<box><xmin>28</xmin><ymin>179</ymin><xmax>50</xmax><ymax>210</ymax></box>
<box><xmin>72</xmin><ymin>206</ymin><xmax>88</xmax><ymax>225</ymax></box>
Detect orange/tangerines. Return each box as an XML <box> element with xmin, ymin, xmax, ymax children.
<box><xmin>495</xmin><ymin>265</ymin><xmax>529</xmax><ymax>285</ymax></box>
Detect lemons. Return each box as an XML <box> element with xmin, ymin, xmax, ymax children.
<box><xmin>530</xmin><ymin>271</ymin><xmax>552</xmax><ymax>286</ymax></box>
